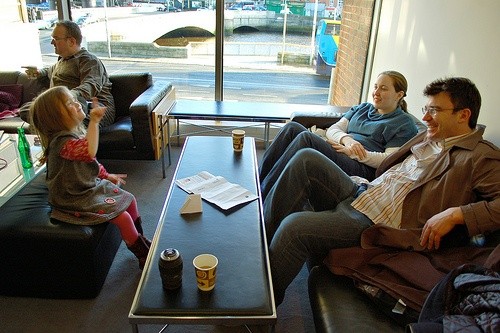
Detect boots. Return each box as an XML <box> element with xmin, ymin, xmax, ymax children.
<box><xmin>126</xmin><ymin>216</ymin><xmax>151</xmax><ymax>270</ymax></box>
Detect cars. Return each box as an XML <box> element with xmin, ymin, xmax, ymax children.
<box><xmin>229</xmin><ymin>4</ymin><xmax>267</xmax><ymax>11</ymax></box>
<box><xmin>150</xmin><ymin>3</ymin><xmax>177</xmax><ymax>12</ymax></box>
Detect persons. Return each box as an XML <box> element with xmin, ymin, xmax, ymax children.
<box><xmin>263</xmin><ymin>77</ymin><xmax>500</xmax><ymax>309</ymax></box>
<box><xmin>257</xmin><ymin>71</ymin><xmax>419</xmax><ymax>204</ymax></box>
<box><xmin>20</xmin><ymin>20</ymin><xmax>116</xmax><ymax>130</ymax></box>
<box><xmin>29</xmin><ymin>85</ymin><xmax>152</xmax><ymax>271</ymax></box>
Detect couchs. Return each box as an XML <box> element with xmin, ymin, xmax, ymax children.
<box><xmin>0</xmin><ymin>71</ymin><xmax>176</xmax><ymax>180</ymax></box>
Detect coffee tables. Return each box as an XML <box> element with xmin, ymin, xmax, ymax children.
<box><xmin>127</xmin><ymin>135</ymin><xmax>277</xmax><ymax>333</ymax></box>
<box><xmin>0</xmin><ymin>133</ymin><xmax>48</xmax><ymax>208</ymax></box>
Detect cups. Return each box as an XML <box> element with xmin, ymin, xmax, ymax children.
<box><xmin>232</xmin><ymin>129</ymin><xmax>245</xmax><ymax>152</ymax></box>
<box><xmin>28</xmin><ymin>67</ymin><xmax>37</xmax><ymax>80</ymax></box>
<box><xmin>193</xmin><ymin>254</ymin><xmax>218</xmax><ymax>291</ymax></box>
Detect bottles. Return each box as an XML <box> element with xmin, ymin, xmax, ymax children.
<box><xmin>158</xmin><ymin>248</ymin><xmax>184</xmax><ymax>291</ymax></box>
<box><xmin>17</xmin><ymin>126</ymin><xmax>33</xmax><ymax>168</ymax></box>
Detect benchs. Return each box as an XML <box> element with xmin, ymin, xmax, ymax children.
<box><xmin>290</xmin><ymin>110</ymin><xmax>500</xmax><ymax>333</ymax></box>
<box><xmin>0</xmin><ymin>168</ymin><xmax>123</xmax><ymax>302</ymax></box>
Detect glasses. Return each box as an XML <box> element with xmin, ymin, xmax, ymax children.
<box><xmin>51</xmin><ymin>36</ymin><xmax>72</xmax><ymax>41</ymax></box>
<box><xmin>422</xmin><ymin>105</ymin><xmax>454</xmax><ymax>115</ymax></box>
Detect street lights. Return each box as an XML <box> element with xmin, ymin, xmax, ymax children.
<box><xmin>281</xmin><ymin>3</ymin><xmax>291</xmax><ymax>65</ymax></box>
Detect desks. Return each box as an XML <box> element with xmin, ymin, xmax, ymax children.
<box><xmin>165</xmin><ymin>101</ymin><xmax>351</xmax><ymax>165</ymax></box>
